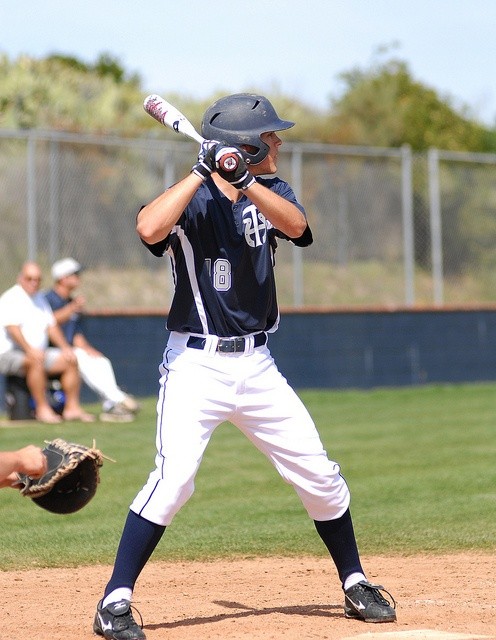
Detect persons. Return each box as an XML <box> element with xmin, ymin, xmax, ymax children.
<box><xmin>43</xmin><ymin>256</ymin><xmax>141</xmax><ymax>423</ymax></box>
<box><xmin>1</xmin><ymin>256</ymin><xmax>97</xmax><ymax>428</ymax></box>
<box><xmin>0</xmin><ymin>444</ymin><xmax>48</xmax><ymax>486</ymax></box>
<box><xmin>92</xmin><ymin>93</ymin><xmax>397</xmax><ymax>636</ymax></box>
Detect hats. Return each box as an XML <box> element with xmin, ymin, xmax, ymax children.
<box><xmin>51</xmin><ymin>257</ymin><xmax>85</xmax><ymax>282</ymax></box>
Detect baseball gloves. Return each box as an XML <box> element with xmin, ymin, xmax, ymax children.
<box><xmin>17</xmin><ymin>439</ymin><xmax>117</xmax><ymax>514</ymax></box>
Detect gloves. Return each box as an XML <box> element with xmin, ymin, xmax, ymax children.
<box><xmin>213</xmin><ymin>144</ymin><xmax>255</xmax><ymax>191</ymax></box>
<box><xmin>191</xmin><ymin>138</ymin><xmax>229</xmax><ymax>180</ymax></box>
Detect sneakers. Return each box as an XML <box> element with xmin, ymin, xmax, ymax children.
<box><xmin>343</xmin><ymin>581</ymin><xmax>397</xmax><ymax>623</ymax></box>
<box><xmin>93</xmin><ymin>598</ymin><xmax>146</xmax><ymax>640</ymax></box>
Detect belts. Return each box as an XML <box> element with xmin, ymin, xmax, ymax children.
<box><xmin>187</xmin><ymin>332</ymin><xmax>266</xmax><ymax>353</ymax></box>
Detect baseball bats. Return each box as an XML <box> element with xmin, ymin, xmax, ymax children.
<box><xmin>143</xmin><ymin>93</ymin><xmax>238</xmax><ymax>171</ymax></box>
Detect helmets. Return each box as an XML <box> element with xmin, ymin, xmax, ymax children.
<box><xmin>200</xmin><ymin>92</ymin><xmax>295</xmax><ymax>165</ymax></box>
